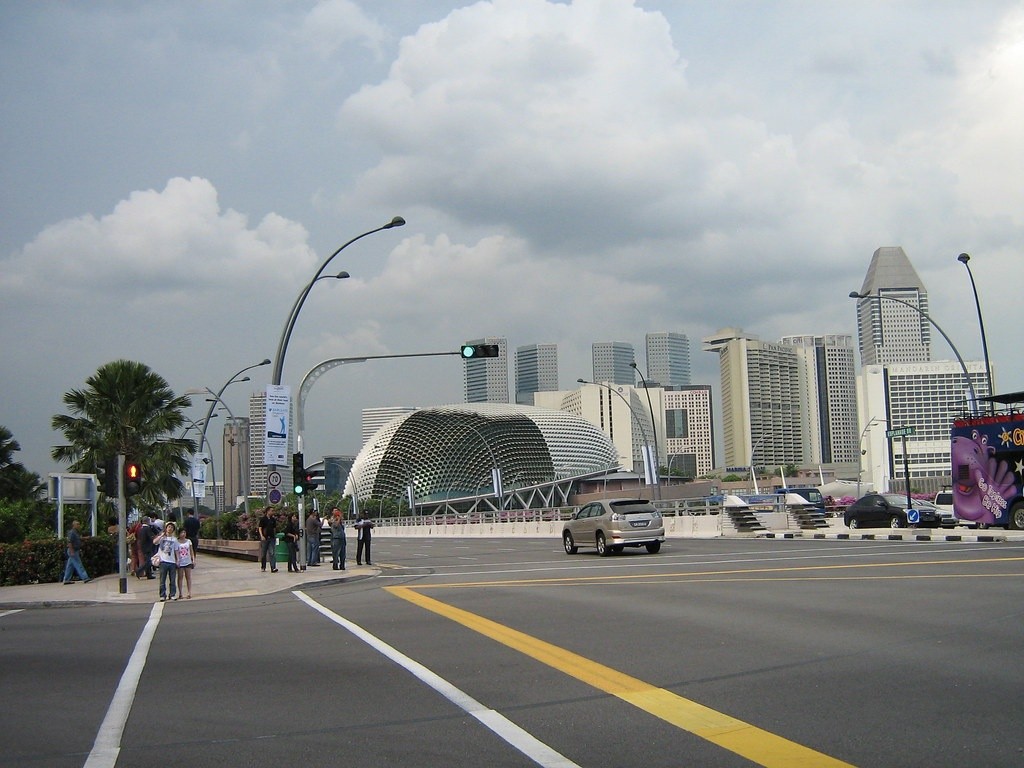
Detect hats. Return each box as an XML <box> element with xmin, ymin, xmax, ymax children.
<box><xmin>335</xmin><ymin>511</ymin><xmax>341</xmax><ymax>516</ymax></box>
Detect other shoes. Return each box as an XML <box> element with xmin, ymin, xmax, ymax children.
<box><xmin>64</xmin><ymin>582</ymin><xmax>75</xmax><ymax>585</ymax></box>
<box><xmin>261</xmin><ymin>567</ymin><xmax>266</xmax><ymax>572</ymax></box>
<box><xmin>147</xmin><ymin>576</ymin><xmax>155</xmax><ymax>579</ymax></box>
<box><xmin>169</xmin><ymin>596</ymin><xmax>177</xmax><ymax>601</ymax></box>
<box><xmin>84</xmin><ymin>578</ymin><xmax>94</xmax><ymax>584</ymax></box>
<box><xmin>271</xmin><ymin>568</ymin><xmax>278</xmax><ymax>573</ymax></box>
<box><xmin>160</xmin><ymin>597</ymin><xmax>166</xmax><ymax>601</ymax></box>
<box><xmin>137</xmin><ymin>576</ymin><xmax>140</xmax><ymax>580</ymax></box>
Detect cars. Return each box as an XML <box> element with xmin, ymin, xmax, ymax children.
<box><xmin>844</xmin><ymin>485</ymin><xmax>989</xmax><ymax>530</ymax></box>
<box><xmin>562</xmin><ymin>499</ymin><xmax>666</xmax><ymax>557</ymax></box>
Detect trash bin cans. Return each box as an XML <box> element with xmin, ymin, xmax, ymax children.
<box><xmin>275</xmin><ymin>533</ymin><xmax>288</xmax><ymax>563</ymax></box>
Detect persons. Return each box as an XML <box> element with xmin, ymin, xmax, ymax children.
<box><xmin>175</xmin><ymin>528</ymin><xmax>197</xmax><ymax>599</ymax></box>
<box><xmin>284</xmin><ymin>513</ymin><xmax>299</xmax><ymax>572</ymax></box>
<box><xmin>152</xmin><ymin>523</ymin><xmax>180</xmax><ymax>601</ymax></box>
<box><xmin>108</xmin><ymin>509</ymin><xmax>200</xmax><ymax>579</ymax></box>
<box><xmin>259</xmin><ymin>506</ymin><xmax>278</xmax><ymax>572</ymax></box>
<box><xmin>306</xmin><ymin>506</ymin><xmax>347</xmax><ymax>570</ymax></box>
<box><xmin>355</xmin><ymin>509</ymin><xmax>373</xmax><ymax>565</ymax></box>
<box><xmin>63</xmin><ymin>520</ymin><xmax>94</xmax><ymax>585</ymax></box>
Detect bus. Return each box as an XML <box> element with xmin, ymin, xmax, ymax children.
<box><xmin>948</xmin><ymin>391</ymin><xmax>1024</xmax><ymax>530</ymax></box>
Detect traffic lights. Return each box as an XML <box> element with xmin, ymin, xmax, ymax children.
<box><xmin>293</xmin><ymin>454</ymin><xmax>304</xmax><ymax>495</ymax></box>
<box><xmin>97</xmin><ymin>461</ymin><xmax>106</xmax><ymax>492</ymax></box>
<box><xmin>123</xmin><ymin>463</ymin><xmax>141</xmax><ymax>495</ymax></box>
<box><xmin>306</xmin><ymin>470</ymin><xmax>318</xmax><ymax>495</ymax></box>
<box><xmin>461</xmin><ymin>345</ymin><xmax>499</xmax><ymax>358</ymax></box>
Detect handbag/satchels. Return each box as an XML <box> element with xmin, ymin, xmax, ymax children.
<box><xmin>151</xmin><ymin>553</ymin><xmax>160</xmax><ymax>567</ymax></box>
<box><xmin>258</xmin><ymin>528</ymin><xmax>268</xmax><ymax>539</ymax></box>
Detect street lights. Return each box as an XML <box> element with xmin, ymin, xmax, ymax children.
<box><xmin>323</xmin><ymin>417</ymin><xmax>890</xmax><ymax>519</ymax></box>
<box><xmin>330</xmin><ymin>462</ymin><xmax>360</xmax><ymax>519</ymax></box>
<box><xmin>460</xmin><ymin>412</ymin><xmax>506</xmax><ymax>510</ymax></box>
<box><xmin>184</xmin><ymin>414</ymin><xmax>221</xmax><ymax>539</ymax></box>
<box><xmin>577</xmin><ymin>362</ymin><xmax>662</xmax><ymax>500</ymax></box>
<box><xmin>267</xmin><ymin>215</ymin><xmax>406</xmax><ymax>506</ymax></box>
<box><xmin>194</xmin><ymin>359</ymin><xmax>272</xmax><ymax>520</ymax></box>
<box><xmin>374</xmin><ymin>445</ymin><xmax>416</xmax><ymax>516</ymax></box>
<box><xmin>848</xmin><ymin>252</ymin><xmax>995</xmax><ymax>415</ymax></box>
<box><xmin>205</xmin><ymin>386</ymin><xmax>254</xmax><ymax>540</ymax></box>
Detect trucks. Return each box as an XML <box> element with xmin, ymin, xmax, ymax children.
<box><xmin>703</xmin><ymin>487</ymin><xmax>825</xmax><ymax>523</ymax></box>
